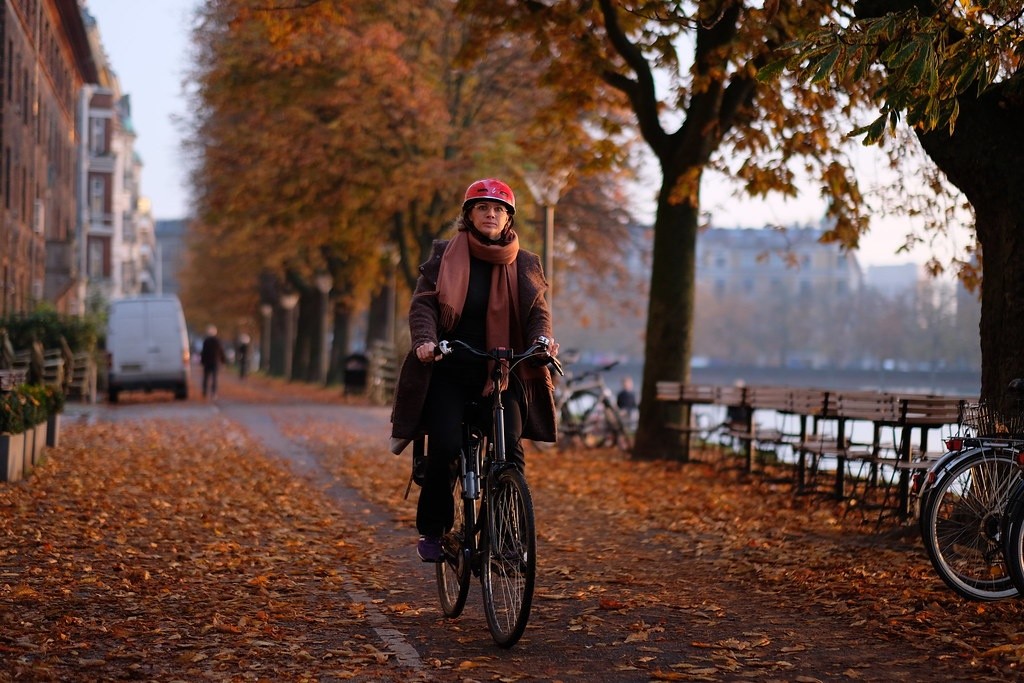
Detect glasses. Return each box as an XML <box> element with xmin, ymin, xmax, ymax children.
<box><xmin>472</xmin><ymin>202</ymin><xmax>508</xmax><ymax>215</ymax></box>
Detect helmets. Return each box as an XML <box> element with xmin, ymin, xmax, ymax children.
<box><xmin>462</xmin><ymin>178</ymin><xmax>516</xmax><ymax>216</ymax></box>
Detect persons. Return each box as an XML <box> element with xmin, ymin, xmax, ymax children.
<box><xmin>388</xmin><ymin>178</ymin><xmax>558</xmax><ymax>575</ymax></box>
<box><xmin>198</xmin><ymin>324</ymin><xmax>228</xmax><ymax>400</ymax></box>
<box><xmin>236</xmin><ymin>334</ymin><xmax>253</xmax><ymax>378</ymax></box>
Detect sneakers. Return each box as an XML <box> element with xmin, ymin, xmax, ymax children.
<box><xmin>416</xmin><ymin>533</ymin><xmax>445</xmax><ymax>563</ymax></box>
<box><xmin>477</xmin><ymin>539</ymin><xmax>527</xmax><ymax>578</ymax></box>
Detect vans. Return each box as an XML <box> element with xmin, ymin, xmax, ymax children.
<box><xmin>101</xmin><ymin>291</ymin><xmax>192</xmax><ymax>405</ymax></box>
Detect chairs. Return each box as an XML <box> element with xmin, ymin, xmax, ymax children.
<box><xmin>653</xmin><ymin>382</ymin><xmax>981</xmax><ymax>529</ymax></box>
<box><xmin>0</xmin><ymin>330</ymin><xmax>99</xmax><ymax>402</ymax></box>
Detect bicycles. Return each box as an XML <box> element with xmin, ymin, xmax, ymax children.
<box><xmin>998</xmin><ymin>479</ymin><xmax>1024</xmax><ymax>597</ymax></box>
<box><xmin>922</xmin><ymin>433</ymin><xmax>1024</xmax><ymax>602</ymax></box>
<box><xmin>916</xmin><ymin>378</ymin><xmax>1024</xmax><ymax>592</ymax></box>
<box><xmin>530</xmin><ymin>351</ymin><xmax>633</xmax><ymax>458</ymax></box>
<box><xmin>406</xmin><ymin>334</ymin><xmax>565</xmax><ymax>649</ymax></box>
